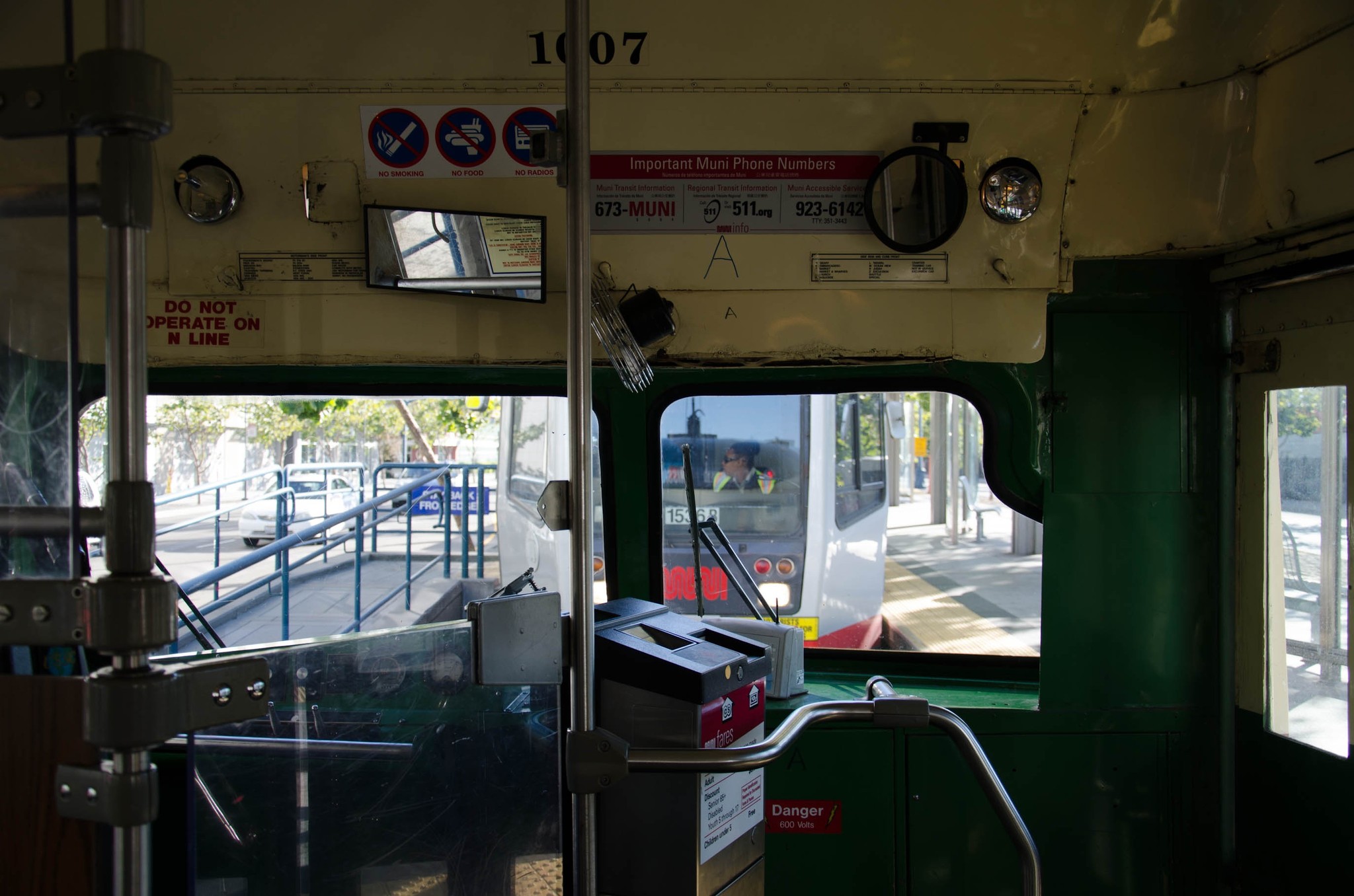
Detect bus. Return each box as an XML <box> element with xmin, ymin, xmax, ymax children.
<box><xmin>495</xmin><ymin>396</ymin><xmax>907</xmax><ymax>652</ymax></box>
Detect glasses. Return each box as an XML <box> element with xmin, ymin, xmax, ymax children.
<box><xmin>723</xmin><ymin>455</ymin><xmax>747</xmax><ymax>464</ymax></box>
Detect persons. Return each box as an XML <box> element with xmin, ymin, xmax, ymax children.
<box><xmin>714</xmin><ymin>440</ymin><xmax>776</xmax><ymax>494</ymax></box>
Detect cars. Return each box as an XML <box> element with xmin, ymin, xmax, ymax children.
<box><xmin>237</xmin><ymin>473</ymin><xmax>365</xmax><ymax>547</ymax></box>
<box><xmin>391</xmin><ymin>467</ymin><xmax>442</xmax><ymax>509</ymax></box>
